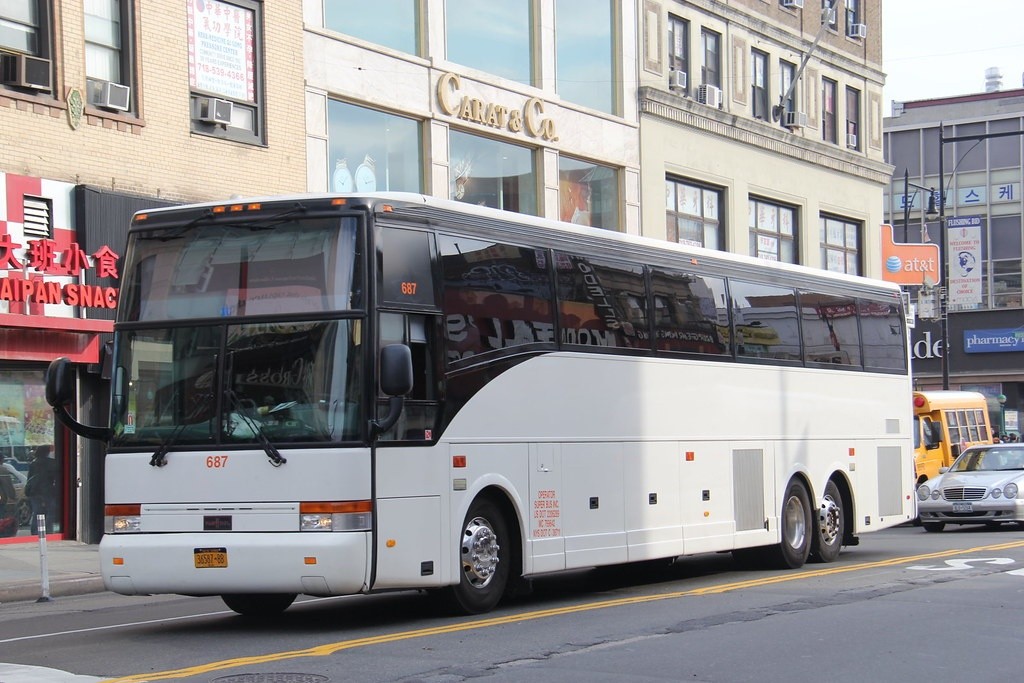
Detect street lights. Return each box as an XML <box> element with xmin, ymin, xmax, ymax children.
<box><xmin>904</xmin><ymin>182</ymin><xmax>938</xmax><ymax>292</ymax></box>
<box><xmin>997</xmin><ymin>394</ymin><xmax>1007</xmax><ymax>434</ymax></box>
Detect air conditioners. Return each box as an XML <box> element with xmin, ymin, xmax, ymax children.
<box><xmin>718</xmin><ymin>90</ymin><xmax>722</xmax><ymax>104</ymax></box>
<box><xmin>193</xmin><ymin>96</ymin><xmax>234</xmax><ymax>125</ymax></box>
<box><xmin>93</xmin><ymin>81</ymin><xmax>130</xmax><ymax>111</ymax></box>
<box><xmin>784</xmin><ymin>0</ymin><xmax>803</xmax><ymax>9</ymax></box>
<box><xmin>822</xmin><ymin>8</ymin><xmax>835</xmax><ymax>24</ymax></box>
<box><xmin>850</xmin><ymin>24</ymin><xmax>866</xmax><ymax>39</ymax></box>
<box><xmin>785</xmin><ymin>111</ymin><xmax>807</xmax><ymax>128</ymax></box>
<box><xmin>698</xmin><ymin>84</ymin><xmax>720</xmax><ymax>109</ymax></box>
<box><xmin>669</xmin><ymin>70</ymin><xmax>687</xmax><ymax>89</ymax></box>
<box><xmin>847</xmin><ymin>134</ymin><xmax>856</xmax><ymax>147</ymax></box>
<box><xmin>3</xmin><ymin>54</ymin><xmax>52</xmax><ymax>92</ymax></box>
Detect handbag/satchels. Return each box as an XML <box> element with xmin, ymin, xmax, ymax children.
<box><xmin>24</xmin><ymin>473</ymin><xmax>56</xmax><ymax>494</ymax></box>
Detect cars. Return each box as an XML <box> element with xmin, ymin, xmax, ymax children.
<box><xmin>916</xmin><ymin>442</ymin><xmax>1023</xmax><ymax>533</ymax></box>
<box><xmin>2</xmin><ymin>463</ymin><xmax>32</xmax><ymax>526</ymax></box>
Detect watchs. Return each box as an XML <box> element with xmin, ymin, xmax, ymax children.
<box><xmin>334</xmin><ymin>153</ymin><xmax>377</xmax><ymax>193</ymax></box>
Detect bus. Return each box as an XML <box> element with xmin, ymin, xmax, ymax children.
<box><xmin>45</xmin><ymin>187</ymin><xmax>919</xmax><ymax>622</ymax></box>
<box><xmin>912</xmin><ymin>391</ymin><xmax>995</xmax><ymax>488</ymax></box>
<box><xmin>0</xmin><ymin>417</ymin><xmax>33</xmax><ymax>485</ymax></box>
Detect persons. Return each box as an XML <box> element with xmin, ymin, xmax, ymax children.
<box><xmin>25</xmin><ymin>445</ymin><xmax>57</xmax><ymax>534</ymax></box>
<box><xmin>0</xmin><ymin>454</ymin><xmax>16</xmax><ymax>519</ymax></box>
<box><xmin>991</xmin><ymin>426</ymin><xmax>1018</xmax><ymax>444</ymax></box>
